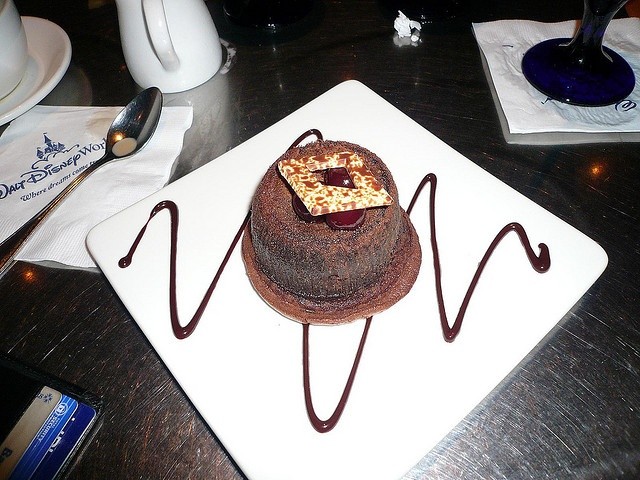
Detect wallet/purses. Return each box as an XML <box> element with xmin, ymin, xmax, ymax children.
<box><xmin>0</xmin><ymin>352</ymin><xmax>104</xmax><ymax>480</ymax></box>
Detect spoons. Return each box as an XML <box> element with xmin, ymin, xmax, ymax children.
<box><xmin>1</xmin><ymin>86</ymin><xmax>163</xmax><ymax>281</ymax></box>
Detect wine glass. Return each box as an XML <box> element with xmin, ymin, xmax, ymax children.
<box><xmin>519</xmin><ymin>0</ymin><xmax>635</xmax><ymax>107</ymax></box>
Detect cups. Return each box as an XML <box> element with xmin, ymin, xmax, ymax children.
<box><xmin>1</xmin><ymin>0</ymin><xmax>29</xmax><ymax>98</ymax></box>
<box><xmin>115</xmin><ymin>0</ymin><xmax>224</xmax><ymax>94</ymax></box>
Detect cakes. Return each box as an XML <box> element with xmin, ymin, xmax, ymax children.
<box><xmin>241</xmin><ymin>140</ymin><xmax>422</xmax><ymax>324</ymax></box>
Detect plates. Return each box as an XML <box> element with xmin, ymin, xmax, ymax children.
<box><xmin>1</xmin><ymin>15</ymin><xmax>73</xmax><ymax>127</ymax></box>
<box><xmin>86</xmin><ymin>79</ymin><xmax>610</xmax><ymax>480</ymax></box>
